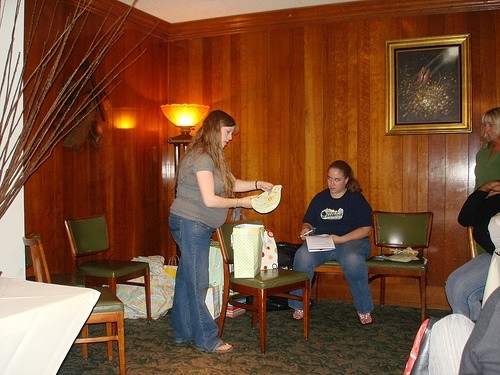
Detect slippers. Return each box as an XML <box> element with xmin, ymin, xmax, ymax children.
<box><xmin>214</xmin><ymin>341</ymin><xmax>235</xmax><ymax>353</ymax></box>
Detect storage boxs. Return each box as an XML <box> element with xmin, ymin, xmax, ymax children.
<box><xmin>226</xmin><ymin>289</ymin><xmax>246</xmax><ymax>319</ymax></box>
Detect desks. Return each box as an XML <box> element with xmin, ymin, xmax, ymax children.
<box><xmin>0</xmin><ymin>277</ymin><xmax>101</xmax><ymax>375</ymax></box>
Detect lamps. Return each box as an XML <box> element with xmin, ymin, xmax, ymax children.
<box><xmin>161</xmin><ymin>104</ymin><xmax>211</xmax><ymax>266</ymax></box>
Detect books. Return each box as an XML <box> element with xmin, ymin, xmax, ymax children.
<box><xmin>306</xmin><ymin>235</ymin><xmax>335</xmax><ymax>253</ymax></box>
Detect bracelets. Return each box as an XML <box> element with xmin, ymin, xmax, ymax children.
<box><xmin>234</xmin><ymin>199</ymin><xmax>238</xmax><ymax>208</ymax></box>
<box><xmin>255</xmin><ymin>180</ymin><xmax>258</xmax><ymax>189</ymax></box>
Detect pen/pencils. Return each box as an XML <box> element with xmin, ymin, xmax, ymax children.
<box><xmin>299</xmin><ymin>227</ymin><xmax>317</xmax><ymax>238</ymax></box>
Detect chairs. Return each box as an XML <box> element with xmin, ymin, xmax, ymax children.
<box><xmin>21</xmin><ymin>215</ymin><xmax>154</xmax><ymax>375</ymax></box>
<box><xmin>215</xmin><ymin>210</ymin><xmax>489</xmax><ymax>375</ymax></box>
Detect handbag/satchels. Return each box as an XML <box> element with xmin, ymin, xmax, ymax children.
<box><xmin>231</xmin><ymin>223</ymin><xmax>264</xmax><ymax>279</ymax></box>
<box><xmin>261</xmin><ymin>230</ymin><xmax>278</xmax><ymax>270</ymax></box>
<box><xmin>163</xmin><ymin>255</ymin><xmax>180</xmax><ymax>279</ymax></box>
<box><xmin>204</xmin><ymin>284</ymin><xmax>220</xmax><ymax>320</ymax></box>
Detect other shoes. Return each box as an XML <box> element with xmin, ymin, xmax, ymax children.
<box><xmin>357</xmin><ymin>312</ymin><xmax>373</xmax><ymax>325</ymax></box>
<box><xmin>293</xmin><ymin>309</ymin><xmax>304</xmax><ymax>320</ymax></box>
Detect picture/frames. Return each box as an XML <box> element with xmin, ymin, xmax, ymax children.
<box><xmin>383</xmin><ymin>34</ymin><xmax>473</xmax><ymax>136</ymax></box>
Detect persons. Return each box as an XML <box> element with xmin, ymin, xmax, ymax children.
<box><xmin>428</xmin><ymin>285</ymin><xmax>500</xmax><ymax>375</ymax></box>
<box><xmin>169</xmin><ymin>110</ymin><xmax>274</xmax><ymax>353</ymax></box>
<box><xmin>288</xmin><ymin>160</ymin><xmax>374</xmax><ymax>325</ymax></box>
<box><xmin>445</xmin><ymin>181</ymin><xmax>500</xmax><ymax>322</ymax></box>
<box><xmin>475</xmin><ymin>107</ymin><xmax>500</xmax><ymax>193</ymax></box>
